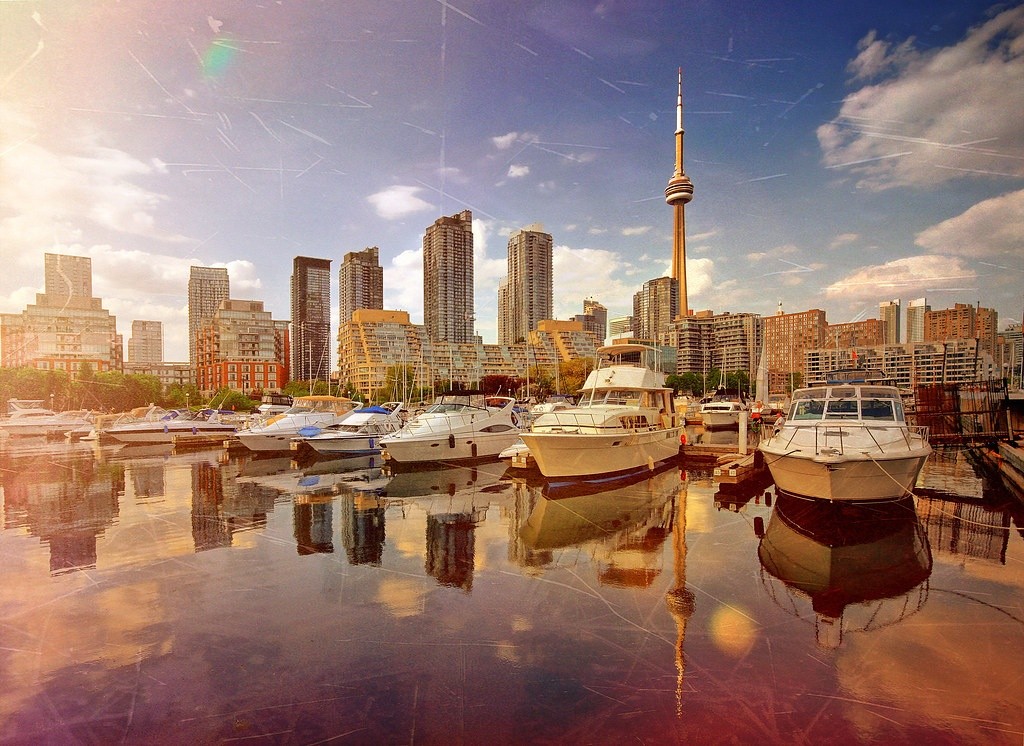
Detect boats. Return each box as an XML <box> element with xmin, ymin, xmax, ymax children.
<box><xmin>378</xmin><ymin>387</ymin><xmax>529</xmax><ymax>463</ymax></box>
<box><xmin>0</xmin><ymin>397</ymin><xmax>104</xmax><ymax>440</ymax></box>
<box><xmin>105</xmin><ymin>406</ymin><xmax>237</xmax><ymax>445</ymax></box>
<box><xmin>292</xmin><ymin>400</ymin><xmax>409</xmax><ymax>455</ymax></box>
<box><xmin>514</xmin><ymin>458</ymin><xmax>690</xmax><ymax>570</ymax></box>
<box><xmin>518</xmin><ymin>343</ymin><xmax>685</xmax><ymax>477</ymax></box>
<box><xmin>234</xmin><ymin>396</ymin><xmax>364</xmax><ymax>454</ymax></box>
<box><xmin>499</xmin><ymin>434</ymin><xmax>535</xmax><ymax>466</ymax></box>
<box><xmin>235</xmin><ymin>456</ymin><xmax>519</xmax><ymax>515</ymax></box>
<box><xmin>754</xmin><ymin>489</ymin><xmax>935</xmax><ymax>647</ymax></box>
<box><xmin>759</xmin><ymin>369</ymin><xmax>932</xmax><ymax>502</ymax></box>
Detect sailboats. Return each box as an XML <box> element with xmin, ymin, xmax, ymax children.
<box><xmin>295</xmin><ymin>325</ymin><xmax>789</xmax><ymax>431</ymax></box>
